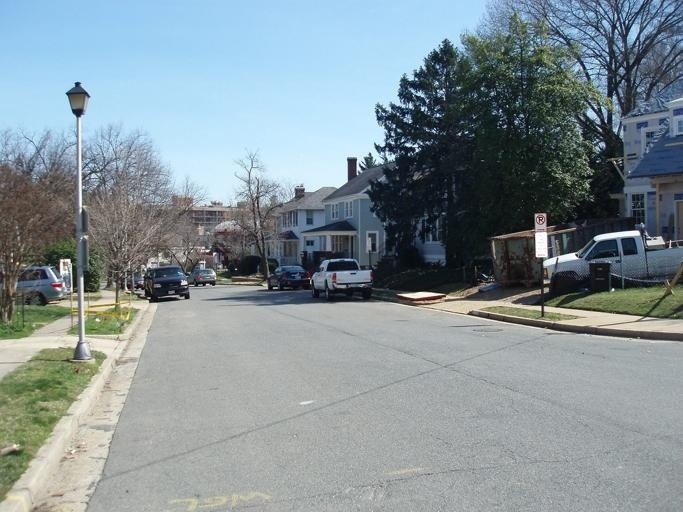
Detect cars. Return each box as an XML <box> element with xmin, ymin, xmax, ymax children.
<box><xmin>121</xmin><ymin>265</ymin><xmax>190</xmax><ymax>300</ymax></box>
<box><xmin>188</xmin><ymin>268</ymin><xmax>217</xmax><ymax>287</ymax></box>
<box><xmin>268</xmin><ymin>265</ymin><xmax>313</xmax><ymax>290</ymax></box>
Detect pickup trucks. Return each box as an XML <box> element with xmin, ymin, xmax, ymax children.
<box><xmin>310</xmin><ymin>258</ymin><xmax>375</xmax><ymax>301</ymax></box>
<box><xmin>542</xmin><ymin>229</ymin><xmax>683</xmax><ymax>293</ymax></box>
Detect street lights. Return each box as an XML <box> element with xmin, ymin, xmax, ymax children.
<box><xmin>65</xmin><ymin>82</ymin><xmax>96</xmax><ymax>364</ymax></box>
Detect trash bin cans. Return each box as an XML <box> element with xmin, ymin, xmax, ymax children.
<box><xmin>589</xmin><ymin>262</ymin><xmax>611</xmax><ymax>292</ymax></box>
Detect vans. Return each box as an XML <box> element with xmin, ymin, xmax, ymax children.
<box><xmin>0</xmin><ymin>266</ymin><xmax>67</xmax><ymax>306</ymax></box>
